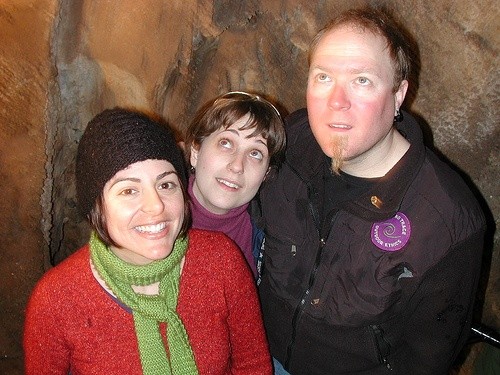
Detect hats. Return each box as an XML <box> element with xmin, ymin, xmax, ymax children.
<box><xmin>76</xmin><ymin>105</ymin><xmax>187</xmax><ymax>218</ymax></box>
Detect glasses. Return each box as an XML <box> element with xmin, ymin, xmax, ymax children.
<box><xmin>191</xmin><ymin>89</ymin><xmax>286</xmax><ymax>138</ymax></box>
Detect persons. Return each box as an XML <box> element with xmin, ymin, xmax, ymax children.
<box><xmin>179</xmin><ymin>89</ymin><xmax>288</xmax><ymax>287</ymax></box>
<box><xmin>249</xmin><ymin>7</ymin><xmax>496</xmax><ymax>375</ymax></box>
<box><xmin>22</xmin><ymin>105</ymin><xmax>276</xmax><ymax>375</ymax></box>
